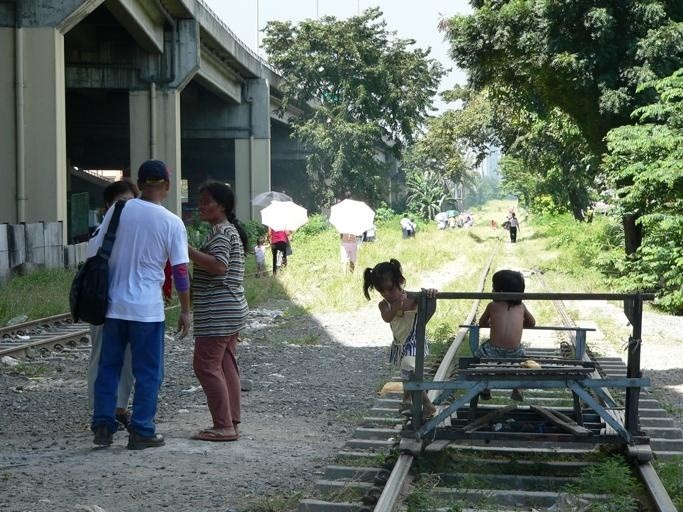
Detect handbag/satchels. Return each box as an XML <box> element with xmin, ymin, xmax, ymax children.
<box><xmin>70</xmin><ymin>200</ymin><xmax>125</xmax><ymax>325</ymax></box>
<box><xmin>286</xmin><ymin>235</ymin><xmax>292</xmax><ymax>256</ymax></box>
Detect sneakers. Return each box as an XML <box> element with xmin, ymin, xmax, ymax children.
<box><xmin>399</xmin><ymin>402</ymin><xmax>413</xmax><ymax>416</ymax></box>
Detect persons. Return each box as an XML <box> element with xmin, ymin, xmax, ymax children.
<box><xmin>90</xmin><ymin>159</ymin><xmax>190</xmax><ymax>451</ymax></box>
<box><xmin>478</xmin><ymin>268</ymin><xmax>537</xmax><ymax>404</ymax></box>
<box><xmin>86</xmin><ymin>179</ymin><xmax>140</xmax><ymax>428</ymax></box>
<box><xmin>365</xmin><ymin>219</ymin><xmax>378</xmax><ymax>243</ymax></box>
<box><xmin>438</xmin><ymin>216</ymin><xmax>472</xmax><ymax>230</ymax></box>
<box><xmin>491</xmin><ymin>220</ymin><xmax>497</xmax><ymax>230</ymax></box>
<box><xmin>409</xmin><ymin>218</ymin><xmax>416</xmax><ymax>238</ymax></box>
<box><xmin>186</xmin><ymin>179</ymin><xmax>251</xmax><ymax>443</ymax></box>
<box><xmin>399</xmin><ymin>213</ymin><xmax>412</xmax><ymax>239</ymax></box>
<box><xmin>363</xmin><ymin>258</ymin><xmax>439</xmax><ymax>423</ymax></box>
<box><xmin>507</xmin><ymin>212</ymin><xmax>520</xmax><ymax>243</ymax></box>
<box><xmin>339</xmin><ymin>232</ymin><xmax>358</xmax><ymax>281</ymax></box>
<box><xmin>253</xmin><ymin>225</ymin><xmax>292</xmax><ymax>278</ymax></box>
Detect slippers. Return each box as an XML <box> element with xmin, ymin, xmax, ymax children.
<box><xmin>192</xmin><ymin>429</ymin><xmax>237</xmax><ymax>440</ymax></box>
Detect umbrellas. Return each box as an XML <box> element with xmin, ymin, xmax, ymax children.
<box><xmin>258</xmin><ymin>200</ymin><xmax>310</xmax><ymax>234</ymax></box>
<box><xmin>445</xmin><ymin>210</ymin><xmax>459</xmax><ymax>217</ymax></box>
<box><xmin>434</xmin><ymin>212</ymin><xmax>448</xmax><ymax>221</ymax></box>
<box><xmin>328</xmin><ymin>198</ymin><xmax>377</xmax><ymax>236</ymax></box>
<box><xmin>251</xmin><ymin>191</ymin><xmax>294</xmax><ymax>207</ymax></box>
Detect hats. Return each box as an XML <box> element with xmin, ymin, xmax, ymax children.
<box><xmin>138</xmin><ymin>160</ymin><xmax>173</xmax><ymax>180</ymax></box>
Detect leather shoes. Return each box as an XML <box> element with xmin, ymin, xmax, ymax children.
<box><xmin>93</xmin><ymin>426</ymin><xmax>113</xmax><ymax>445</ymax></box>
<box><xmin>127</xmin><ymin>432</ymin><xmax>165</xmax><ymax>450</ymax></box>
